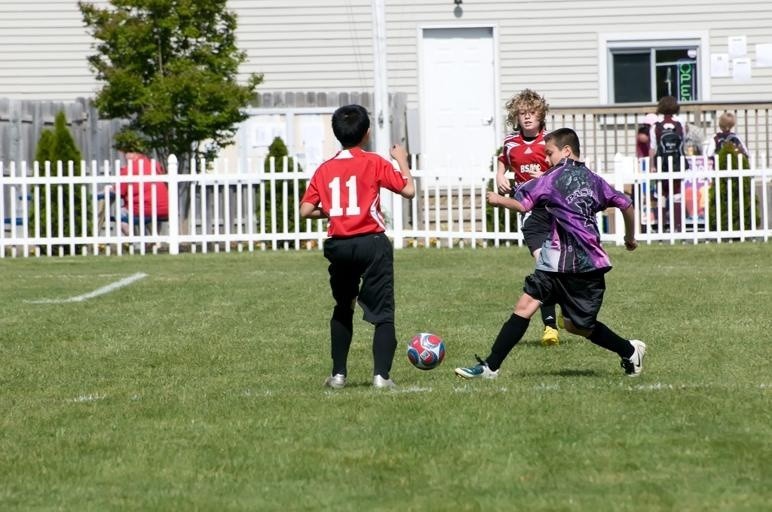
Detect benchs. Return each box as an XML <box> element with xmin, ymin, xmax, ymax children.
<box><xmin>112</xmin><ymin>215</ymin><xmax>167</xmax><ymax>251</ymax></box>
<box><xmin>4</xmin><ymin>218</ymin><xmax>26</xmax><ymax>225</ymax></box>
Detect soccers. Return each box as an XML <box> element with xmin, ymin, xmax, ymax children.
<box><xmin>407</xmin><ymin>333</ymin><xmax>445</xmax><ymax>370</ymax></box>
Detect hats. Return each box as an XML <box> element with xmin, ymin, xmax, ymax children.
<box><xmin>113</xmin><ymin>140</ymin><xmax>140</xmax><ymax>152</ymax></box>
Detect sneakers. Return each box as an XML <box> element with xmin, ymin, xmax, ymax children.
<box><xmin>372</xmin><ymin>374</ymin><xmax>397</xmax><ymax>389</ymax></box>
<box><xmin>540</xmin><ymin>324</ymin><xmax>560</xmax><ymax>346</ymax></box>
<box><xmin>454</xmin><ymin>360</ymin><xmax>499</xmax><ymax>380</ymax></box>
<box><xmin>620</xmin><ymin>339</ymin><xmax>647</xmax><ymax>378</ymax></box>
<box><xmin>325</xmin><ymin>373</ymin><xmax>346</xmax><ymax>390</ymax></box>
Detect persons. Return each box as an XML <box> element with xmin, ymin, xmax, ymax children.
<box><xmin>452</xmin><ymin>127</ymin><xmax>648</xmax><ymax>381</ymax></box>
<box><xmin>495</xmin><ymin>88</ymin><xmax>569</xmax><ymax>346</ymax></box>
<box><xmin>635</xmin><ymin>113</ymin><xmax>660</xmax><ymax>233</ymax></box>
<box><xmin>706</xmin><ymin>111</ymin><xmax>751</xmax><ymax>174</ymax></box>
<box><xmin>647</xmin><ymin>96</ymin><xmax>689</xmax><ymax>234</ymax></box>
<box><xmin>297</xmin><ymin>103</ymin><xmax>417</xmax><ymax>394</ymax></box>
<box><xmin>105</xmin><ymin>140</ymin><xmax>169</xmax><ymax>252</ymax></box>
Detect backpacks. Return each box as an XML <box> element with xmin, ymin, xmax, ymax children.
<box><xmin>654</xmin><ymin>122</ymin><xmax>690</xmax><ymax>172</ymax></box>
<box><xmin>717</xmin><ymin>132</ymin><xmax>736</xmax><ymax>150</ymax></box>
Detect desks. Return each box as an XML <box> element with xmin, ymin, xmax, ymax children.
<box><xmin>19</xmin><ymin>191</ymin><xmax>116</xmax><ymax>254</ymax></box>
